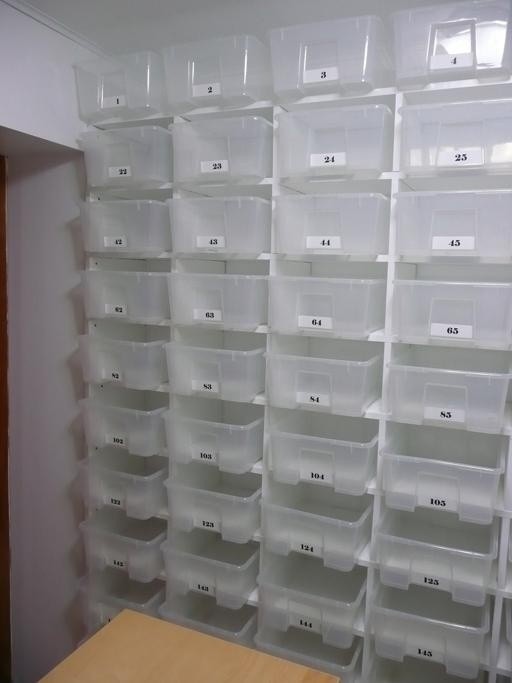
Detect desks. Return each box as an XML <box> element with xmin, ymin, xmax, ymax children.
<box><xmin>37</xmin><ymin>609</ymin><xmax>340</xmax><ymax>683</ymax></box>
